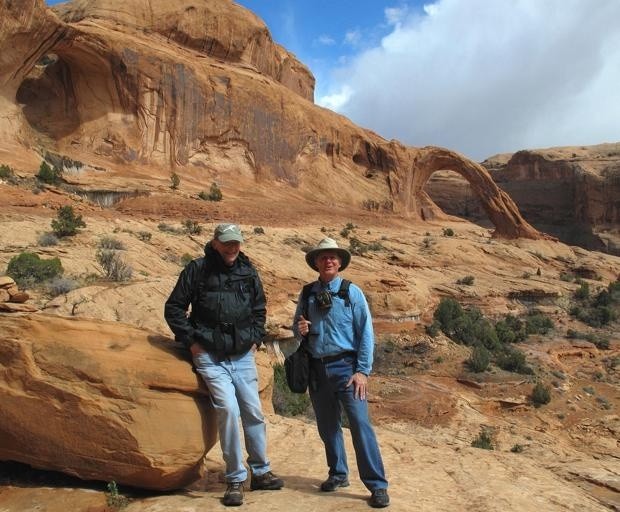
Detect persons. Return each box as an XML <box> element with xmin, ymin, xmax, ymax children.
<box><xmin>294</xmin><ymin>251</ymin><xmax>391</xmax><ymax>507</ymax></box>
<box><xmin>165</xmin><ymin>224</ymin><xmax>284</xmax><ymax>506</ymax></box>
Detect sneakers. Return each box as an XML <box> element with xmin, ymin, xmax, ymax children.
<box><xmin>224</xmin><ymin>471</ymin><xmax>284</xmax><ymax>506</ymax></box>
<box><xmin>321</xmin><ymin>475</ymin><xmax>349</xmax><ymax>491</ymax></box>
<box><xmin>369</xmin><ymin>489</ymin><xmax>390</xmax><ymax>507</ymax></box>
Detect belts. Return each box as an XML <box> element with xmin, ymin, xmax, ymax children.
<box><xmin>320</xmin><ymin>352</ymin><xmax>350</xmax><ymax>363</ymax></box>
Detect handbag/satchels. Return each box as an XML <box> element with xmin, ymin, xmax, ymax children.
<box><xmin>288</xmin><ymin>347</ymin><xmax>310</xmax><ymax>393</ymax></box>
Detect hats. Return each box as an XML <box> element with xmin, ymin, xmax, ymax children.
<box><xmin>214</xmin><ymin>224</ymin><xmax>244</xmax><ymax>243</ymax></box>
<box><xmin>305</xmin><ymin>238</ymin><xmax>351</xmax><ymax>272</ymax></box>
